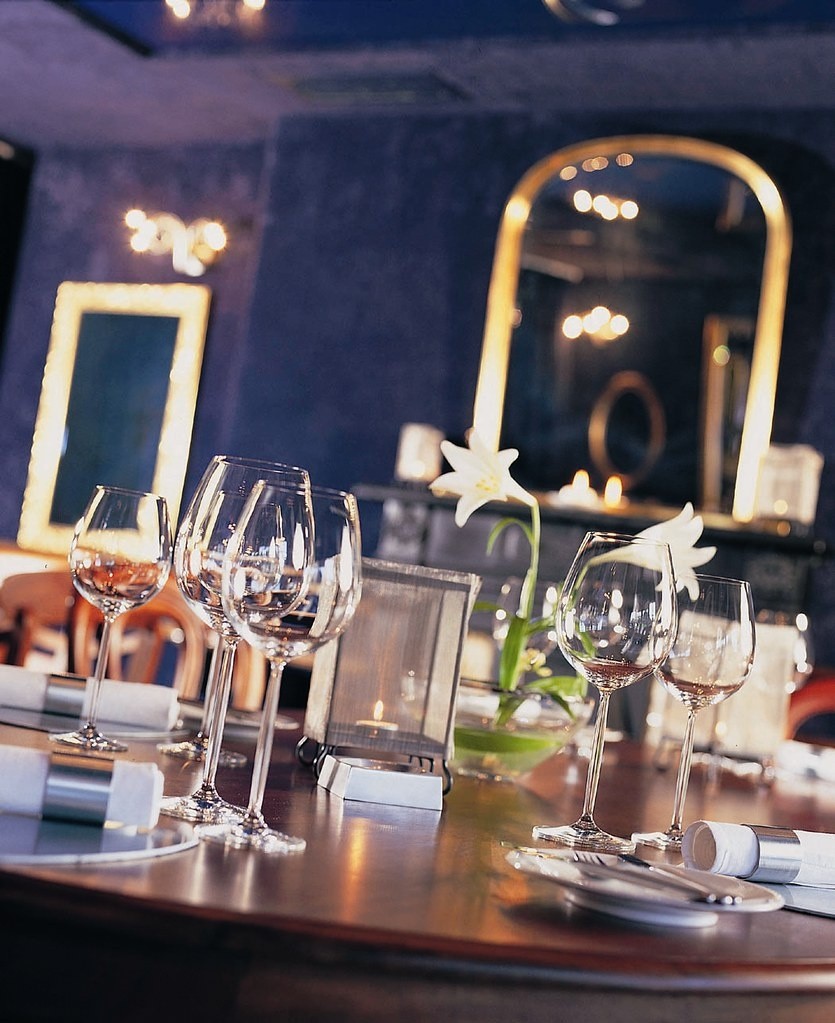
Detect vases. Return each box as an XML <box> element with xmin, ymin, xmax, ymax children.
<box><xmin>448</xmin><ymin>679</ymin><xmax>588</xmax><ymax>783</ymax></box>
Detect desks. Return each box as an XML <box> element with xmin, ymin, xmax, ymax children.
<box><xmin>350</xmin><ymin>484</ymin><xmax>835</xmax><ymax>743</ymax></box>
<box><xmin>0</xmin><ymin>699</ymin><xmax>835</xmax><ymax>1023</ymax></box>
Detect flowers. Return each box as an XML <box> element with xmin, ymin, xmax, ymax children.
<box><xmin>427</xmin><ymin>429</ymin><xmax>718</xmax><ymax>772</ymax></box>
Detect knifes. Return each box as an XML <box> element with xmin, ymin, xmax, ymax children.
<box><xmin>617</xmin><ymin>853</ymin><xmax>743</xmax><ymax>906</ymax></box>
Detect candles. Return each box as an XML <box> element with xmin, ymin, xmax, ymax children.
<box><xmin>355</xmin><ymin>700</ymin><xmax>399</xmax><ymax>731</ymax></box>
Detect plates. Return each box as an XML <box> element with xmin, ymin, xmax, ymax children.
<box><xmin>504</xmin><ymin>847</ymin><xmax>786</xmax><ymax>927</ymax></box>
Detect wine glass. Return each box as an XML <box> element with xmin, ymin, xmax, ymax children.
<box><xmin>531</xmin><ymin>531</ymin><xmax>680</xmax><ymax>852</ymax></box>
<box><xmin>160</xmin><ymin>455</ymin><xmax>314</xmax><ymax>820</ymax></box>
<box><xmin>630</xmin><ymin>573</ymin><xmax>757</xmax><ymax>856</ymax></box>
<box><xmin>193</xmin><ymin>479</ymin><xmax>365</xmax><ymax>856</ymax></box>
<box><xmin>47</xmin><ymin>485</ymin><xmax>175</xmax><ymax>752</ymax></box>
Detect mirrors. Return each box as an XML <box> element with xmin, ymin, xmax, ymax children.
<box><xmin>473</xmin><ymin>136</ymin><xmax>794</xmax><ymax>528</ymax></box>
<box><xmin>15</xmin><ymin>283</ymin><xmax>213</xmax><ymax>562</ymax></box>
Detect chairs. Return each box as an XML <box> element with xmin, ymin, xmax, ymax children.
<box><xmin>0</xmin><ymin>571</ymin><xmax>318</xmax><ymax>712</ymax></box>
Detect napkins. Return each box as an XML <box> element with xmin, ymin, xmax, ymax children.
<box><xmin>0</xmin><ymin>745</ymin><xmax>164</xmax><ymax>831</ymax></box>
<box><xmin>681</xmin><ymin>820</ymin><xmax>835</xmax><ymax>889</ymax></box>
<box><xmin>0</xmin><ymin>665</ymin><xmax>178</xmax><ymax>730</ymax></box>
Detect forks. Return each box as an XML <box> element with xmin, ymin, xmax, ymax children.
<box><xmin>572</xmin><ymin>850</ymin><xmax>718</xmax><ymax>904</ymax></box>
